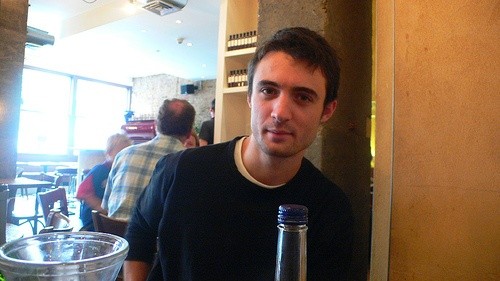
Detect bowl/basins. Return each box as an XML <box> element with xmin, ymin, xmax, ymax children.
<box><xmin>0</xmin><ymin>231</ymin><xmax>128</xmax><ymax>281</ymax></box>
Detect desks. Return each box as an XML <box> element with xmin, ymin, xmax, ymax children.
<box><xmin>0</xmin><ymin>177</ymin><xmax>53</xmax><ymax>247</ymax></box>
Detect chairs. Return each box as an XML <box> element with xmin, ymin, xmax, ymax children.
<box><xmin>0</xmin><ymin>164</ymin><xmax>132</xmax><ymax>238</ymax></box>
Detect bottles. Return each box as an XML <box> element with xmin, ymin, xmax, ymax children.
<box><xmin>275</xmin><ymin>205</ymin><xmax>309</xmax><ymax>281</ymax></box>
<box><xmin>227</xmin><ymin>30</ymin><xmax>257</xmax><ymax>87</ymax></box>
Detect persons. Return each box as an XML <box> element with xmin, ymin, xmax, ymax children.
<box><xmin>184</xmin><ymin>130</ymin><xmax>198</xmax><ymax>150</ymax></box>
<box><xmin>199</xmin><ymin>98</ymin><xmax>215</xmax><ymax>146</ymax></box>
<box><xmin>100</xmin><ymin>98</ymin><xmax>196</xmax><ymax>220</ymax></box>
<box><xmin>124</xmin><ymin>27</ymin><xmax>363</xmax><ymax>281</ymax></box>
<box><xmin>77</xmin><ymin>134</ymin><xmax>132</xmax><ymax>226</ymax></box>
